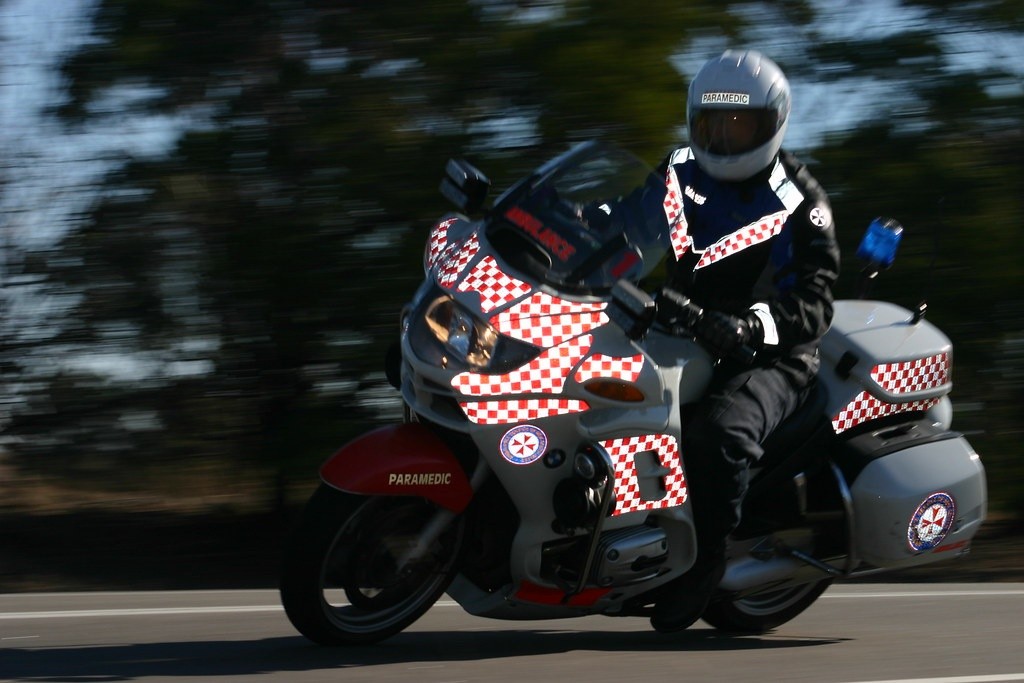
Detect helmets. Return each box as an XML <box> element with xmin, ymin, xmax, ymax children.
<box><xmin>686</xmin><ymin>50</ymin><xmax>792</xmax><ymax>179</ymax></box>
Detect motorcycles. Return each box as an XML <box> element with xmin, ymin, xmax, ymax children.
<box><xmin>280</xmin><ymin>133</ymin><xmax>987</xmax><ymax>648</ymax></box>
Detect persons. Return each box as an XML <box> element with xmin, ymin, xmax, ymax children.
<box><xmin>547</xmin><ymin>46</ymin><xmax>840</xmax><ymax>638</ymax></box>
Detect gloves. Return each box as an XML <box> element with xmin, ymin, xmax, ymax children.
<box><xmin>695</xmin><ymin>309</ymin><xmax>763</xmax><ymax>357</ymax></box>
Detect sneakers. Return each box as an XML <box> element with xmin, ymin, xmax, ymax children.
<box><xmin>650</xmin><ymin>532</ymin><xmax>731</xmax><ymax>632</ymax></box>
